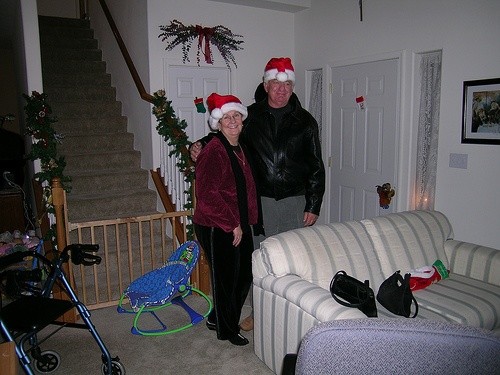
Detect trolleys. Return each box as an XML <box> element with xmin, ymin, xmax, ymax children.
<box><xmin>0</xmin><ymin>244</ymin><xmax>125</xmax><ymax>375</ymax></box>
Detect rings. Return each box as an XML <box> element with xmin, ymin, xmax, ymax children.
<box><xmin>238</xmin><ymin>236</ymin><xmax>240</xmax><ymax>238</ymax></box>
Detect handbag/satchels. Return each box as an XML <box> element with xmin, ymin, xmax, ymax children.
<box><xmin>376</xmin><ymin>270</ymin><xmax>418</xmax><ymax>318</ymax></box>
<box><xmin>330</xmin><ymin>270</ymin><xmax>378</xmax><ymax>318</ymax></box>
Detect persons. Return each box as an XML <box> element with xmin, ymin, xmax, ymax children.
<box><xmin>189</xmin><ymin>57</ymin><xmax>326</xmax><ymax>331</ymax></box>
<box><xmin>192</xmin><ymin>93</ymin><xmax>258</xmax><ymax>346</ymax></box>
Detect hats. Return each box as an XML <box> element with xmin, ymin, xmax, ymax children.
<box><xmin>206</xmin><ymin>92</ymin><xmax>248</xmax><ymax>130</ymax></box>
<box><xmin>264</xmin><ymin>57</ymin><xmax>295</xmax><ymax>84</ymax></box>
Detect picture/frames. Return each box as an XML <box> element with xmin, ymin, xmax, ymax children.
<box><xmin>460</xmin><ymin>78</ymin><xmax>500</xmax><ymax>145</ymax></box>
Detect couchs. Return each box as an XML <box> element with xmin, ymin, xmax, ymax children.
<box><xmin>251</xmin><ymin>209</ymin><xmax>500</xmax><ymax>375</ymax></box>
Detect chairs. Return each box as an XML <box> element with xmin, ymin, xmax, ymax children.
<box><xmin>283</xmin><ymin>318</ymin><xmax>500</xmax><ymax>375</ymax></box>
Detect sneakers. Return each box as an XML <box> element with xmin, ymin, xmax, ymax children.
<box><xmin>206</xmin><ymin>320</ymin><xmax>217</xmax><ymax>330</ymax></box>
<box><xmin>229</xmin><ymin>332</ymin><xmax>249</xmax><ymax>346</ymax></box>
